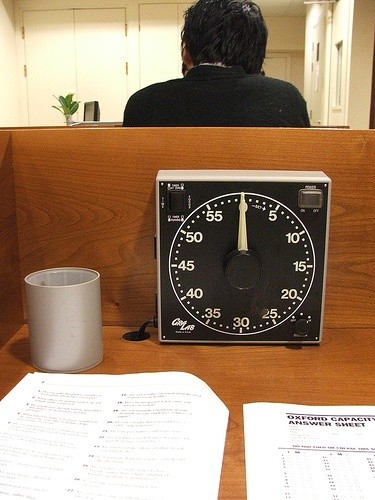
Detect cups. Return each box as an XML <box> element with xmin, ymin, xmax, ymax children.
<box><xmin>23</xmin><ymin>267</ymin><xmax>104</xmax><ymax>373</ymax></box>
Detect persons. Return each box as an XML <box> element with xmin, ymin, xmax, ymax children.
<box><xmin>122</xmin><ymin>0</ymin><xmax>312</xmax><ymax>128</ymax></box>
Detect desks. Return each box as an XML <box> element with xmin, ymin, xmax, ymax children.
<box><xmin>0</xmin><ymin>128</ymin><xmax>375</xmax><ymax>500</ymax></box>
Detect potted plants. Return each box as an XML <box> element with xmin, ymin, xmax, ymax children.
<box><xmin>51</xmin><ymin>93</ymin><xmax>82</xmax><ymax>126</ymax></box>
